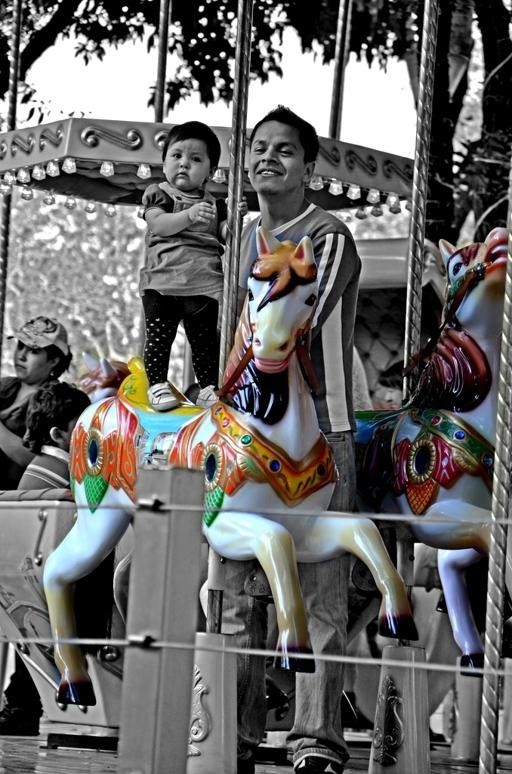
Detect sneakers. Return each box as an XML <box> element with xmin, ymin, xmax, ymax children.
<box><xmin>196</xmin><ymin>385</ymin><xmax>219</xmax><ymax>408</ymax></box>
<box><xmin>295</xmin><ymin>757</ymin><xmax>342</xmax><ymax>774</ymax></box>
<box><xmin>147</xmin><ymin>382</ymin><xmax>179</xmax><ymax>411</ymax></box>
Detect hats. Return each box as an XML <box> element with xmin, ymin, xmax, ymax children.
<box><xmin>7</xmin><ymin>317</ymin><xmax>69</xmax><ymax>357</ymax></box>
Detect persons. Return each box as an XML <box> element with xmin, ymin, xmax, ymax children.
<box><xmin>0</xmin><ymin>314</ymin><xmax>91</xmax><ymax>490</ymax></box>
<box><xmin>138</xmin><ymin>120</ymin><xmax>249</xmax><ymax>411</ymax></box>
<box><xmin>221</xmin><ymin>104</ymin><xmax>362</xmax><ymax>773</ymax></box>
<box><xmin>376</xmin><ymin>360</ymin><xmax>404</xmax><ymax>412</ymax></box>
<box><xmin>41</xmin><ymin>224</ymin><xmax>420</xmax><ymax>707</ymax></box>
<box><xmin>1</xmin><ymin>381</ymin><xmax>98</xmax><ymax>735</ymax></box>
